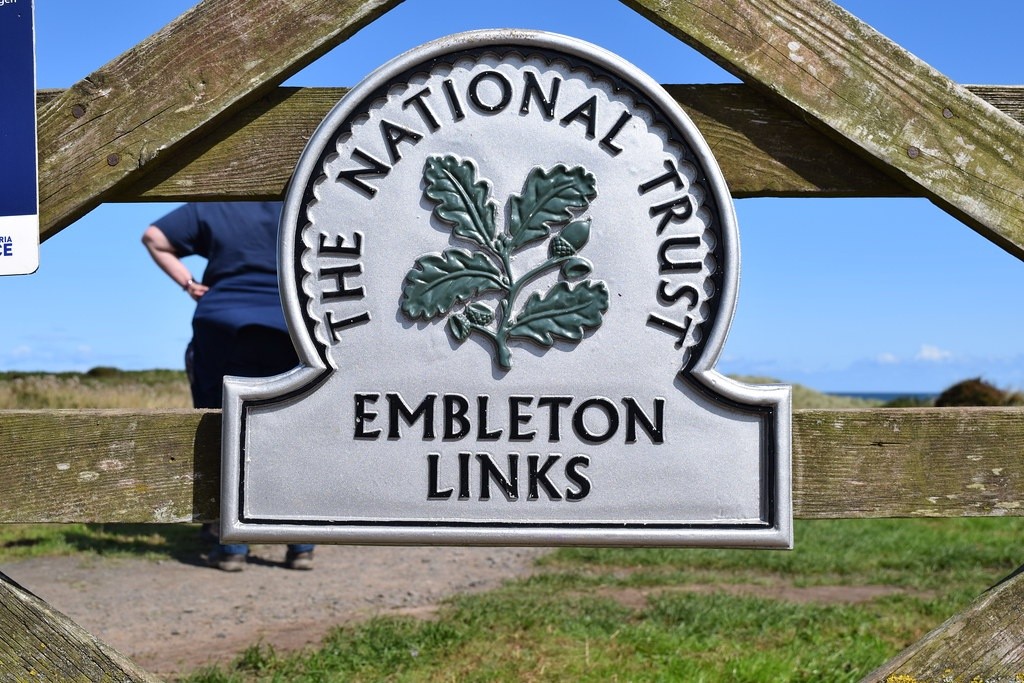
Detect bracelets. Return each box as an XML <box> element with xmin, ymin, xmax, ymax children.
<box><xmin>183</xmin><ymin>278</ymin><xmax>196</xmax><ymax>290</ymax></box>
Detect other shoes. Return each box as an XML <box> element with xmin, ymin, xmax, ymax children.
<box><xmin>285</xmin><ymin>550</ymin><xmax>314</xmax><ymax>569</ymax></box>
<box><xmin>207</xmin><ymin>549</ymin><xmax>245</xmax><ymax>571</ymax></box>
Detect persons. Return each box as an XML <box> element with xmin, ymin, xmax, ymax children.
<box><xmin>142</xmin><ymin>201</ymin><xmax>315</xmax><ymax>571</ymax></box>
